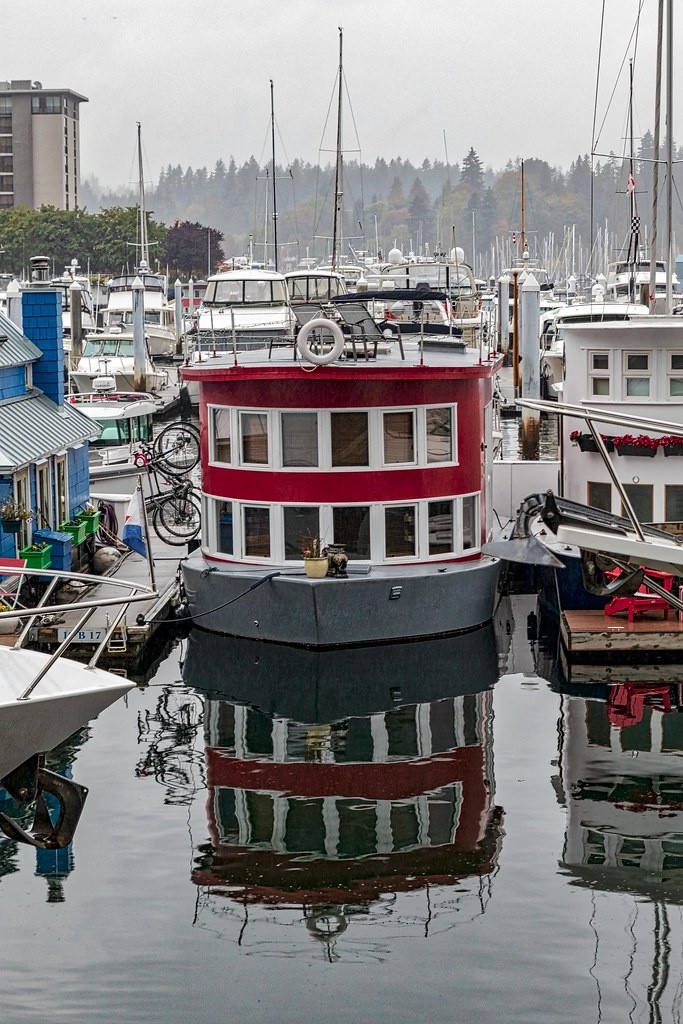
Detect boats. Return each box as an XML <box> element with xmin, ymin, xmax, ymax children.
<box><xmin>0</xmin><ymin>1</ymin><xmax>683</xmax><ymax>787</ymax></box>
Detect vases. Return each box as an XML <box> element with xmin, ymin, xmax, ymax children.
<box><xmin>75</xmin><ymin>510</ymin><xmax>101</xmax><ymax>534</ymax></box>
<box><xmin>1</xmin><ymin>519</ymin><xmax>21</xmax><ymax>533</ymax></box>
<box><xmin>19</xmin><ymin>545</ymin><xmax>53</xmax><ymax>569</ymax></box>
<box><xmin>578</xmin><ymin>434</ymin><xmax>615</xmax><ymax>454</ymax></box>
<box><xmin>663</xmin><ymin>445</ymin><xmax>683</xmax><ymax>458</ymax></box>
<box><xmin>59</xmin><ymin>521</ymin><xmax>88</xmax><ymax>546</ymax></box>
<box><xmin>616</xmin><ymin>445</ymin><xmax>658</xmax><ymax>458</ymax></box>
<box><xmin>304</xmin><ymin>558</ymin><xmax>329</xmax><ymax>579</ymax></box>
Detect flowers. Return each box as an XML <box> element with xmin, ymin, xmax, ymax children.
<box><xmin>570</xmin><ymin>431</ymin><xmax>608</xmax><ymax>447</ymax></box>
<box><xmin>79</xmin><ymin>505</ymin><xmax>94</xmax><ymax>516</ymax></box>
<box><xmin>611</xmin><ymin>434</ymin><xmax>683</xmax><ymax>450</ymax></box>
<box><xmin>66</xmin><ymin>519</ymin><xmax>82</xmax><ymax>526</ymax></box>
<box><xmin>0</xmin><ymin>495</ymin><xmax>36</xmax><ymax>523</ymax></box>
<box><xmin>300</xmin><ymin>527</ymin><xmax>329</xmax><ymax>559</ymax></box>
<box><xmin>31</xmin><ymin>541</ymin><xmax>47</xmax><ymax>551</ymax></box>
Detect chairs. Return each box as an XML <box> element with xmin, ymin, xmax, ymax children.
<box><xmin>604</xmin><ymin>564</ymin><xmax>674</xmax><ymax>622</ymax></box>
<box><xmin>603</xmin><ymin>681</ymin><xmax>676</xmax><ymax>728</ymax></box>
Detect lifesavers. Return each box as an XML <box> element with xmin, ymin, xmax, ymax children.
<box><xmin>296</xmin><ymin>316</ymin><xmax>343</xmax><ymax>366</ymax></box>
<box><xmin>308</xmin><ymin>910</ymin><xmax>348</xmax><ymax>943</ymax></box>
